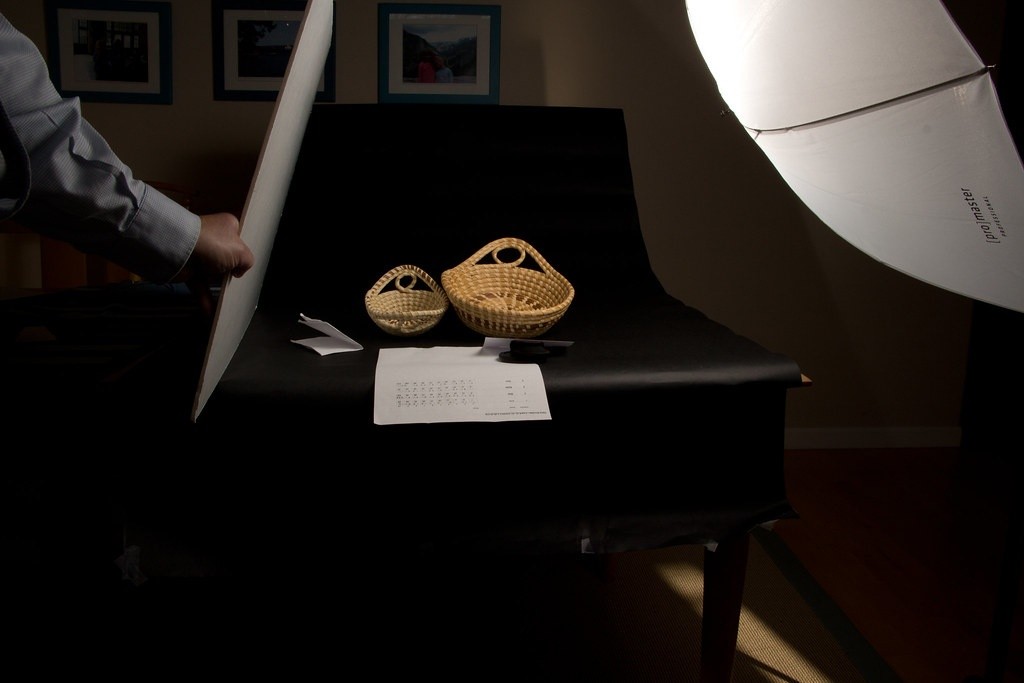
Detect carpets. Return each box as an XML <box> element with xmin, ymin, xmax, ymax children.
<box><xmin>750</xmin><ymin>447</ymin><xmax>1024</xmax><ymax>683</ymax></box>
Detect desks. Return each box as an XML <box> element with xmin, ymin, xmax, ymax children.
<box><xmin>196</xmin><ymin>104</ymin><xmax>814</xmax><ymax>683</ymax></box>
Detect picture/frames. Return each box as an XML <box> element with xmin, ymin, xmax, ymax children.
<box><xmin>212</xmin><ymin>0</ymin><xmax>336</xmax><ymax>102</ymax></box>
<box><xmin>377</xmin><ymin>3</ymin><xmax>501</xmax><ymax>105</ymax></box>
<box><xmin>44</xmin><ymin>0</ymin><xmax>173</xmax><ymax>105</ymax></box>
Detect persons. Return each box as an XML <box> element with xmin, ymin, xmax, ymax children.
<box><xmin>92</xmin><ymin>39</ymin><xmax>125</xmax><ymax>80</ymax></box>
<box><xmin>418</xmin><ymin>48</ymin><xmax>453</xmax><ymax>83</ymax></box>
<box><xmin>0</xmin><ymin>18</ymin><xmax>253</xmax><ymax>276</ymax></box>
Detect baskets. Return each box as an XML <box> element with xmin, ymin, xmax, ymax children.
<box><xmin>364</xmin><ymin>265</ymin><xmax>449</xmax><ymax>335</ymax></box>
<box><xmin>440</xmin><ymin>237</ymin><xmax>575</xmax><ymax>338</ymax></box>
